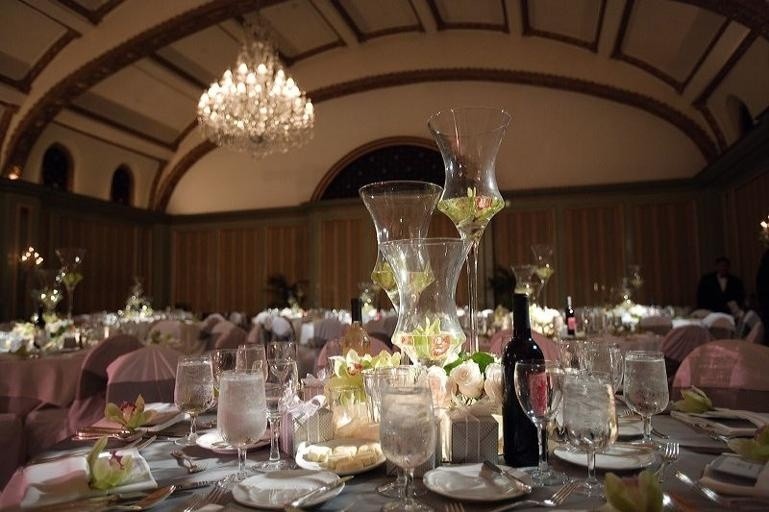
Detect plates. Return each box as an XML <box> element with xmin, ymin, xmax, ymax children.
<box><xmin>420</xmin><ymin>463</ymin><xmax>534</xmax><ymax>501</ymax></box>
<box><xmin>199</xmin><ymin>426</ymin><xmax>272</xmax><ymax>455</ymax></box>
<box><xmin>232</xmin><ymin>469</ymin><xmax>347</xmax><ymax>506</ymax></box>
<box><xmin>550</xmin><ymin>441</ymin><xmax>656</xmax><ymax>469</ymax></box>
<box><xmin>615</xmin><ymin>415</ymin><xmax>653</xmax><ymax>437</ymax></box>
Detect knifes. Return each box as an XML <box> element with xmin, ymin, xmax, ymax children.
<box><xmin>283</xmin><ymin>475</ymin><xmax>355</xmax><ymax>512</ymax></box>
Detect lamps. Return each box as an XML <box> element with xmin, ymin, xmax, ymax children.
<box><xmin>193</xmin><ymin>1</ymin><xmax>318</xmax><ymax>159</ymax></box>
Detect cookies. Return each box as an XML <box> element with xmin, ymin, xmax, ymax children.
<box><xmin>307</xmin><ymin>442</ymin><xmax>382</xmax><ymax>473</ymax></box>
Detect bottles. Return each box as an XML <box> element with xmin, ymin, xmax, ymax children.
<box><xmin>503</xmin><ymin>295</ymin><xmax>545</xmax><ymax>467</ymax></box>
<box><xmin>33</xmin><ymin>305</ymin><xmax>46</xmax><ymax>348</ymax></box>
<box><xmin>342</xmin><ymin>298</ymin><xmax>370</xmax><ymax>356</ymax></box>
<box><xmin>566</xmin><ymin>296</ymin><xmax>576</xmax><ymax>335</ymax></box>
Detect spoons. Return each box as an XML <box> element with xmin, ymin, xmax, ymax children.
<box><xmin>97</xmin><ymin>484</ymin><xmax>177</xmax><ymax>512</ymax></box>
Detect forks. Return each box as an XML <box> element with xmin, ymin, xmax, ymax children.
<box><xmin>168</xmin><ymin>481</ymin><xmax>228</xmax><ymax>512</ymax></box>
<box><xmin>442</xmin><ymin>480</ymin><xmax>577</xmax><ymax>512</ymax></box>
<box><xmin>676</xmin><ymin>471</ymin><xmax>769</xmax><ymax>509</ymax></box>
<box><xmin>655</xmin><ymin>443</ymin><xmax>680</xmax><ymax>482</ymax></box>
<box><xmin>660</xmin><ymin>468</ymin><xmax>769</xmax><ymax>512</ymax></box>
<box><xmin>30</xmin><ymin>430</ymin><xmax>157</xmax><ymax>464</ymax></box>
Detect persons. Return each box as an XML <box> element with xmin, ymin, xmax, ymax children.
<box><xmin>697</xmin><ymin>257</ymin><xmax>744</xmax><ymax>314</ymax></box>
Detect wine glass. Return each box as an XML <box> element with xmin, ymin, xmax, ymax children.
<box><xmin>621</xmin><ymin>348</ymin><xmax>670</xmax><ymax>443</ymax></box>
<box><xmin>376</xmin><ymin>379</ymin><xmax>436</xmax><ymax>499</ymax></box>
<box><xmin>558</xmin><ymin>340</ymin><xmax>625</xmax><ymax>395</ymax></box>
<box><xmin>208</xmin><ymin>339</ymin><xmax>302</xmax><ymax>394</ymax></box>
<box><xmin>177</xmin><ymin>359</ymin><xmax>215</xmax><ymax>446</ymax></box>
<box><xmin>218</xmin><ymin>368</ymin><xmax>268</xmax><ymax>473</ymax></box>
<box><xmin>561</xmin><ymin>371</ymin><xmax>618</xmax><ymax>496</ymax></box>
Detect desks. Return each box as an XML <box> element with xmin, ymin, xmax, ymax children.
<box><xmin>0</xmin><ymin>385</ymin><xmax>769</xmax><ymax>512</ymax></box>
